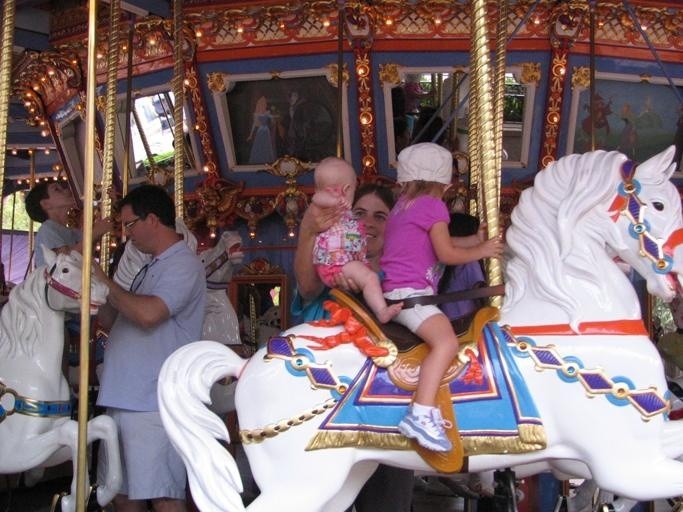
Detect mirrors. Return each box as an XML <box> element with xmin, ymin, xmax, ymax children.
<box><xmin>377</xmin><ymin>61</ymin><xmax>542</xmax><ymax>170</ymax></box>
<box><xmin>96</xmin><ymin>81</ymin><xmax>204</xmax><ymax>186</ymax></box>
<box><xmin>225</xmin><ymin>261</ymin><xmax>288</xmax><ymax>358</ymax></box>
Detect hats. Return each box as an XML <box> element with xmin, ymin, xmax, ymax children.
<box><xmin>396</xmin><ymin>143</ymin><xmax>455</xmax><ymax>184</ymax></box>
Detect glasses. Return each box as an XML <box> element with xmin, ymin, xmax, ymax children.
<box><xmin>124</xmin><ymin>217</ymin><xmax>141</xmax><ymax>230</ymax></box>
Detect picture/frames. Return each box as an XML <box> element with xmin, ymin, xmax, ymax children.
<box><xmin>55</xmin><ymin>101</ymin><xmax>115</xmax><ymax>203</ymax></box>
<box><xmin>206</xmin><ymin>61</ymin><xmax>354</xmax><ymax>178</ymax></box>
<box><xmin>565</xmin><ymin>65</ymin><xmax>683</xmax><ymax>179</ymax></box>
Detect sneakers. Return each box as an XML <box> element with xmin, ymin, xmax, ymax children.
<box><xmin>397</xmin><ymin>409</ymin><xmax>453</xmax><ymax>453</ymax></box>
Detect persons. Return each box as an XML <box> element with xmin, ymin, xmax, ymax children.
<box><xmin>379</xmin><ymin>141</ymin><xmax>507</xmax><ymax>454</ymax></box>
<box><xmin>584</xmin><ymin>94</ymin><xmax>638</xmax><ymax>153</ymax></box>
<box><xmin>398</xmin><ymin>71</ymin><xmax>439</xmax><ymax>151</ymax></box>
<box><xmin>310</xmin><ymin>155</ymin><xmax>404</xmax><ymax>326</ymax></box>
<box><xmin>94</xmin><ymin>182</ymin><xmax>208</xmax><ymax>511</ymax></box>
<box><xmin>438</xmin><ymin>261</ymin><xmax>488</xmax><ymax>324</ymax></box>
<box><xmin>25</xmin><ymin>176</ymin><xmax>117</xmax><ymax>387</ymax></box>
<box><xmin>290</xmin><ymin>184</ymin><xmax>399</xmax><ymax>325</ymax></box>
<box><xmin>245</xmin><ymin>90</ymin><xmax>302</xmax><ymax>167</ymax></box>
